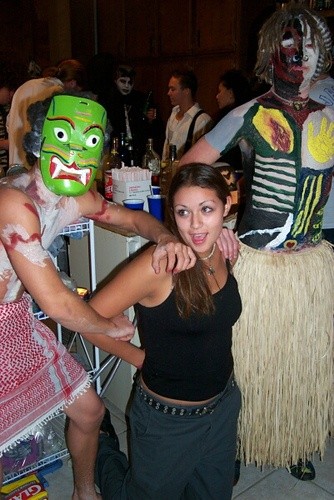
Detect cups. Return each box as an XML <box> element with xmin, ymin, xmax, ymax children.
<box><xmin>147</xmin><ymin>194</ymin><xmax>162</xmax><ymax>221</ymax></box>
<box><xmin>122</xmin><ymin>199</ymin><xmax>144</xmax><ymax>210</ymax></box>
<box><xmin>152</xmin><ymin>186</ymin><xmax>160</xmax><ymax>194</ymax></box>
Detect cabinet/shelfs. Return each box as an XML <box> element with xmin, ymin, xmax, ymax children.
<box><xmin>2</xmin><ymin>219</ymin><xmax>103</xmax><ymax>485</ymax></box>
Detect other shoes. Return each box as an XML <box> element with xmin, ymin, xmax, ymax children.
<box><xmin>286</xmin><ymin>456</ymin><xmax>315</xmax><ymax>480</ymax></box>
<box><xmin>100</xmin><ymin>407</ymin><xmax>117</xmax><ymax>440</ymax></box>
<box><xmin>231</xmin><ymin>457</ymin><xmax>240</xmax><ymax>485</ymax></box>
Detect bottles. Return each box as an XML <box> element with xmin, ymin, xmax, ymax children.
<box><xmin>139</xmin><ymin>90</ymin><xmax>152</xmax><ymax>120</ymax></box>
<box><xmin>103</xmin><ymin>138</ymin><xmax>122</xmax><ymax>202</ymax></box>
<box><xmin>119</xmin><ymin>133</ymin><xmax>135</xmax><ymax>167</ymax></box>
<box><xmin>161</xmin><ymin>145</ymin><xmax>177</xmax><ymax>193</ymax></box>
<box><xmin>141</xmin><ymin>138</ymin><xmax>160</xmax><ymax>185</ymax></box>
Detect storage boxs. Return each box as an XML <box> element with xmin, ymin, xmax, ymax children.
<box><xmin>111</xmin><ymin>179</ymin><xmax>152</xmax><ymax>212</ymax></box>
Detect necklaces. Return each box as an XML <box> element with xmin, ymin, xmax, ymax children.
<box><xmin>270</xmin><ymin>85</ymin><xmax>309</xmax><ymax>110</ymax></box>
<box><xmin>198</xmin><ymin>241</ymin><xmax>216</xmax><ymax>260</ymax></box>
<box><xmin>205</xmin><ymin>255</ymin><xmax>215</xmax><ymax>273</ymax></box>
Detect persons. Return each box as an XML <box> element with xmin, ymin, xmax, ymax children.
<box><xmin>0</xmin><ymin>1</ymin><xmax>334</xmax><ymax>500</ymax></box>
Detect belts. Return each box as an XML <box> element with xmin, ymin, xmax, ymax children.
<box><xmin>134</xmin><ymin>379</ymin><xmax>235</xmax><ymax>416</ymax></box>
